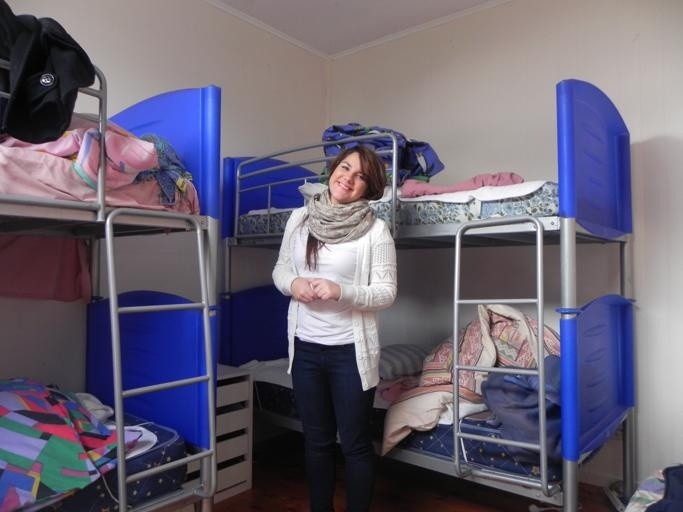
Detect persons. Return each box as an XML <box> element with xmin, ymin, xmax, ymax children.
<box><xmin>270</xmin><ymin>147</ymin><xmax>400</xmax><ymax>511</ymax></box>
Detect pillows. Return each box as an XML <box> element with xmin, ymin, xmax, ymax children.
<box><xmin>378</xmin><ymin>343</ymin><xmax>428</xmax><ymax>379</ymax></box>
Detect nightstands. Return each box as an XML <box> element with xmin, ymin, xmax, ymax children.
<box><xmin>212</xmin><ymin>364</ymin><xmax>253</xmax><ymax>504</ymax></box>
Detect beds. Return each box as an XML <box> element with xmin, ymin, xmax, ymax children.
<box><xmin>220</xmin><ymin>79</ymin><xmax>638</xmax><ymax>512</ymax></box>
<box><xmin>0</xmin><ymin>64</ymin><xmax>221</xmax><ymax>512</ymax></box>
